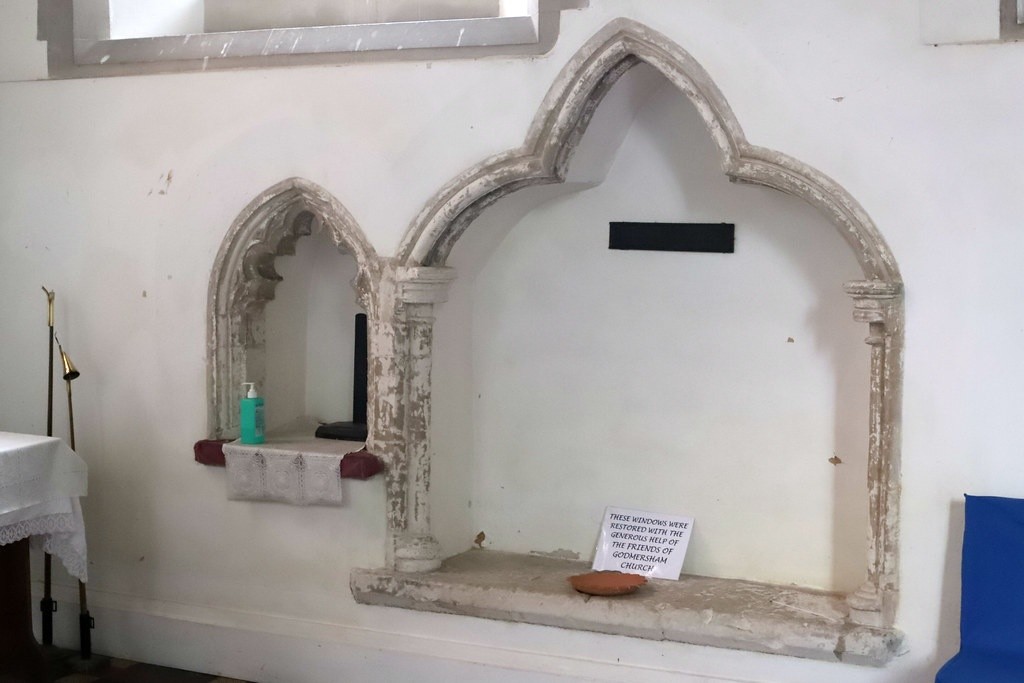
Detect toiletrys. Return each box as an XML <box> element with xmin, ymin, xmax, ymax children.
<box><xmin>239</xmin><ymin>382</ymin><xmax>266</xmax><ymax>445</ymax></box>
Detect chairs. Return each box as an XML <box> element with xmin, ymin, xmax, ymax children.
<box><xmin>932</xmin><ymin>492</ymin><xmax>1024</xmax><ymax>683</ymax></box>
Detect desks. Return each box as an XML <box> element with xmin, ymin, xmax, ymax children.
<box><xmin>0</xmin><ymin>430</ymin><xmax>90</xmax><ymax>683</ymax></box>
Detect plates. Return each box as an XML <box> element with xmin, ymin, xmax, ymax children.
<box><xmin>567</xmin><ymin>570</ymin><xmax>646</xmax><ymax>595</ymax></box>
